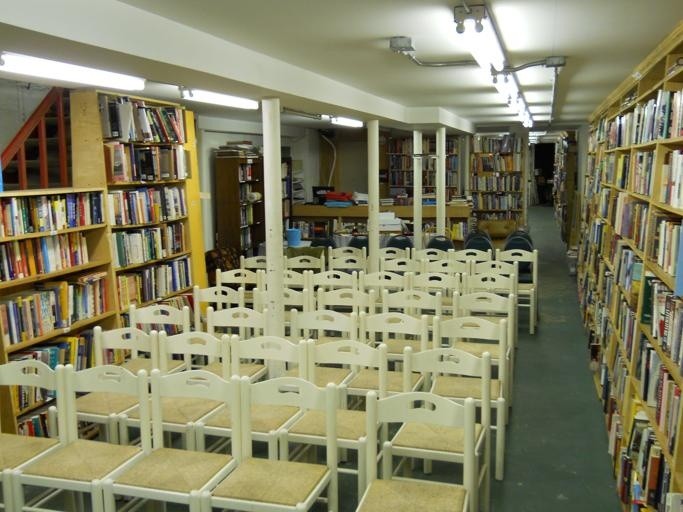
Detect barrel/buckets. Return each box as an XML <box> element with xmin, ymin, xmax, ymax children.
<box><xmin>286</xmin><ymin>228</ymin><xmax>302</xmax><ymax>247</ymax></box>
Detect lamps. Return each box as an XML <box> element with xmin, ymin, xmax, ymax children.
<box><xmin>328</xmin><ymin>116</ymin><xmax>365</xmax><ymax>129</ymax></box>
<box><xmin>451</xmin><ymin>3</ymin><xmax>535</xmax><ymax>130</ymax></box>
<box><xmin>1</xmin><ymin>50</ymin><xmax>148</xmax><ymax>94</ymax></box>
<box><xmin>178</xmin><ymin>85</ymin><xmax>261</xmax><ymax>113</ymax></box>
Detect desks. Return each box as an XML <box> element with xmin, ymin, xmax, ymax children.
<box><xmin>289</xmin><ymin>202</ymin><xmax>469</xmax><ymax>236</ymax></box>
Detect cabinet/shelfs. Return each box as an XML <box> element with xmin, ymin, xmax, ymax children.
<box><xmin>468</xmin><ymin>135</ymin><xmax>532</xmax><ymax>237</ymax></box>
<box><xmin>384</xmin><ymin>138</ymin><xmax>457</xmax><ymax>190</ymax></box>
<box><xmin>277</xmin><ymin>153</ymin><xmax>295</xmax><ymax>232</ymax></box>
<box><xmin>0</xmin><ymin>186</ymin><xmax>121</xmax><ymax>441</ymax></box>
<box><xmin>65</xmin><ymin>87</ymin><xmax>193</xmax><ymax>363</ymax></box>
<box><xmin>214</xmin><ymin>153</ymin><xmax>265</xmax><ymax>258</ymax></box>
<box><xmin>579</xmin><ymin>25</ymin><xmax>683</xmax><ymax>511</ymax></box>
<box><xmin>550</xmin><ymin>131</ymin><xmax>578</xmax><ymax>258</ymax></box>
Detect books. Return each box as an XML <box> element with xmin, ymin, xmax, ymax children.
<box><xmin>213</xmin><ymin>139</ymin><xmax>289</xmax><ymax>253</ymax></box>
<box><xmin>388</xmin><ymin>132</ymin><xmax>526</xmax><ymax>219</ymax></box>
<box><xmin>0</xmin><ymin>190</ymin><xmax>126</xmax><ymax>442</ymax></box>
<box><xmin>576</xmin><ymin>89</ymin><xmax>683</xmax><ymax>512</ymax></box>
<box><xmin>93</xmin><ymin>93</ymin><xmax>194</xmax><ymax>336</ymax></box>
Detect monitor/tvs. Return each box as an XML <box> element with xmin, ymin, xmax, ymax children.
<box><xmin>312</xmin><ymin>186</ymin><xmax>335</xmax><ymax>205</ymax></box>
